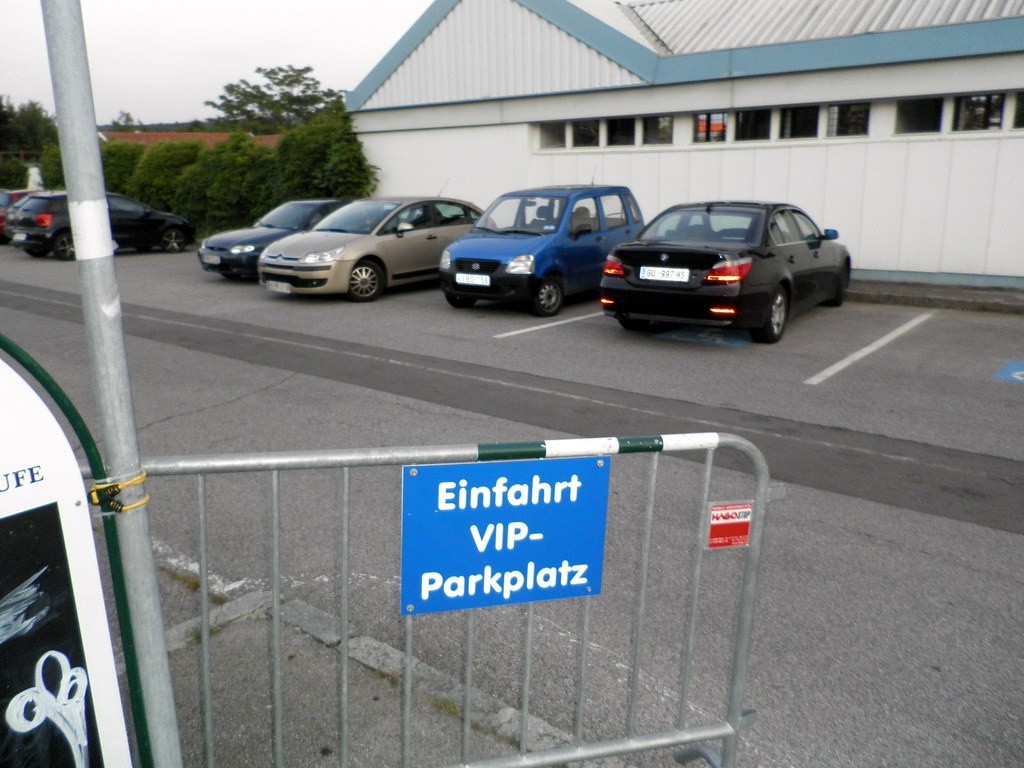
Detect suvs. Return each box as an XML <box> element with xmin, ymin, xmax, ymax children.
<box><xmin>5</xmin><ymin>189</ymin><xmax>195</xmax><ymax>260</ymax></box>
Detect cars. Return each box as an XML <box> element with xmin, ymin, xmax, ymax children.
<box><xmin>0</xmin><ymin>189</ymin><xmax>41</xmax><ymax>241</ymax></box>
<box><xmin>252</xmin><ymin>194</ymin><xmax>493</xmax><ymax>306</ymax></box>
<box><xmin>199</xmin><ymin>202</ymin><xmax>352</xmax><ymax>284</ymax></box>
<box><xmin>438</xmin><ymin>184</ymin><xmax>642</xmax><ymax>318</ymax></box>
<box><xmin>599</xmin><ymin>199</ymin><xmax>852</xmax><ymax>342</ymax></box>
